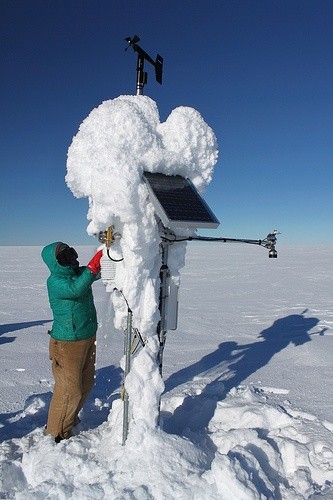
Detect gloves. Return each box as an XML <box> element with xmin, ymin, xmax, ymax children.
<box><xmin>86</xmin><ymin>249</ymin><xmax>102</xmax><ymax>275</ymax></box>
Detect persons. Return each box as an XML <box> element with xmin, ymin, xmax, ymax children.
<box><xmin>41</xmin><ymin>242</ymin><xmax>104</xmax><ymax>443</ymax></box>
